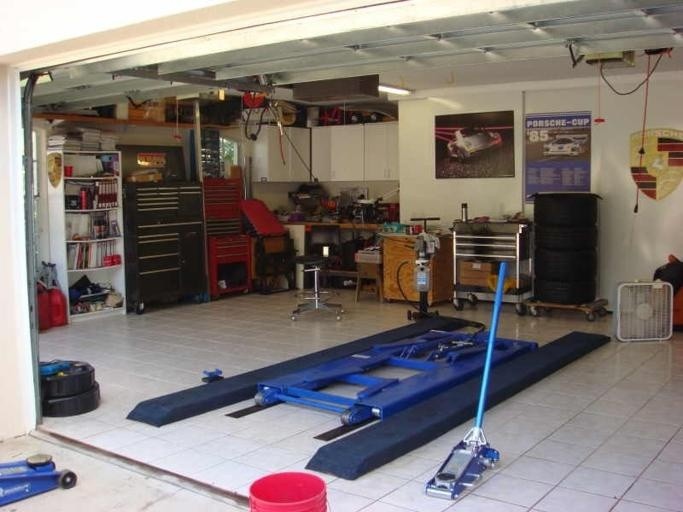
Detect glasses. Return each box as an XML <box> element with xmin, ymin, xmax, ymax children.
<box><xmin>377</xmin><ymin>85</ymin><xmax>412</xmax><ymax>95</ymax></box>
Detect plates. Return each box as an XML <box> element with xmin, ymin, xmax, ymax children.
<box><xmin>46</xmin><ymin>149</ymin><xmax>126</xmax><ymax>324</ymax></box>
<box><xmin>452</xmin><ymin>222</ymin><xmax>532</xmax><ymax>315</ymax></box>
<box><xmin>251</xmin><ymin>122</ymin><xmax>400</xmax><ymax>183</ymax></box>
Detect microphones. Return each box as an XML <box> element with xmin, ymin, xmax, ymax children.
<box><xmin>354</xmin><ymin>253</ymin><xmax>383</xmax><ymax>302</ymax></box>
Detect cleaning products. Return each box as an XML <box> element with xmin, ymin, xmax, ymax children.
<box><xmin>450</xmin><ymin>219</ymin><xmax>534</xmax><ymax>316</ymax></box>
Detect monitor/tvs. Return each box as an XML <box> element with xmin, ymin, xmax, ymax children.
<box><xmin>47</xmin><ymin>128</ymin><xmax>118</xmax><ymax>269</ymax></box>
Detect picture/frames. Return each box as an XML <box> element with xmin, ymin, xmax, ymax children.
<box><xmin>281</xmin><ymin>221</ymin><xmax>381</xmax><ymax>290</ymax></box>
<box><xmin>124</xmin><ymin>180</ymin><xmax>205</xmax><ymax>314</ymax></box>
<box><xmin>383</xmin><ymin>232</ymin><xmax>454</xmax><ymax>306</ymax></box>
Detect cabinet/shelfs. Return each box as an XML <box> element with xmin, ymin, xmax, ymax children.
<box><xmin>94</xmin><ymin>220</ymin><xmax>106</xmax><ymax>239</ymax></box>
<box><xmin>80</xmin><ymin>187</ymin><xmax>88</xmax><ymax>209</ymax></box>
<box><xmin>462</xmin><ymin>204</ymin><xmax>467</xmax><ymax>222</ymax></box>
<box><xmin>417</xmin><ymin>267</ymin><xmax>427</xmax><ymax>291</ymax></box>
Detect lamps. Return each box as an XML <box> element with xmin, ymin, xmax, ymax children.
<box><xmin>290</xmin><ymin>224</ymin><xmax>344</xmax><ymax>321</ymax></box>
<box><xmin>205</xmin><ymin>178</ymin><xmax>253</xmax><ymax>301</ymax></box>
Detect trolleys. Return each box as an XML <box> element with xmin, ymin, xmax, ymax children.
<box><xmin>613</xmin><ymin>278</ymin><xmax>673</xmax><ymax>342</ymax></box>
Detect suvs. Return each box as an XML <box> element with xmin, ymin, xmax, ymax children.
<box><xmin>37</xmin><ymin>283</ymin><xmax>50</xmax><ymax>330</ymax></box>
<box><xmin>250</xmin><ymin>472</ymin><xmax>330</xmax><ymax>512</ymax></box>
<box><xmin>50</xmin><ymin>285</ymin><xmax>67</xmax><ymax>330</ymax></box>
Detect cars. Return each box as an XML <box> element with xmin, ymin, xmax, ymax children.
<box><xmin>104</xmin><ymin>255</ymin><xmax>120</xmax><ymax>266</ymax></box>
<box><xmin>65</xmin><ymin>166</ymin><xmax>72</xmax><ymax>176</ymax></box>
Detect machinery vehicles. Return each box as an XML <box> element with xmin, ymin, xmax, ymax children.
<box><xmin>446</xmin><ymin>126</ymin><xmax>501</xmax><ymax>162</ymax></box>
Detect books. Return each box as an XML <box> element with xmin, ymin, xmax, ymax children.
<box><xmin>460</xmin><ymin>261</ymin><xmax>498</xmax><ymax>287</ymax></box>
